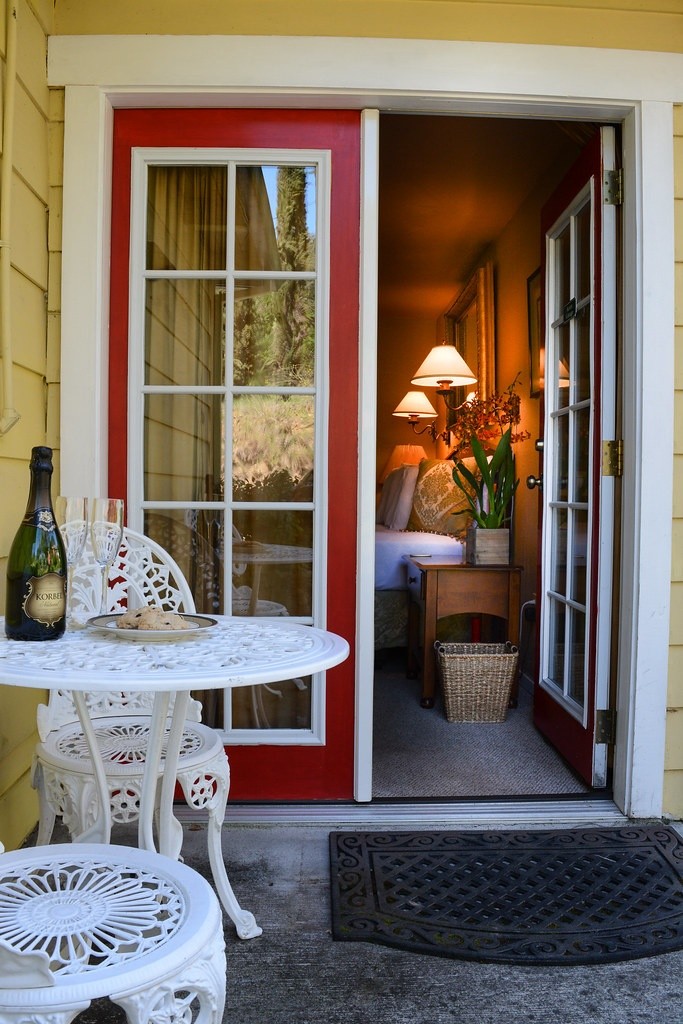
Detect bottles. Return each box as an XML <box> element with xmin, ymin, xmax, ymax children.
<box><xmin>5</xmin><ymin>446</ymin><xmax>67</xmax><ymax>641</ymax></box>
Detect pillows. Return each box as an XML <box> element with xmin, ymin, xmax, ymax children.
<box><xmin>382</xmin><ymin>456</ymin><xmax>493</xmax><ymax>535</ymax></box>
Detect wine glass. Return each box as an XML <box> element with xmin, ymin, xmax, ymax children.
<box><xmin>54</xmin><ymin>497</ymin><xmax>124</xmax><ymax>632</ymax></box>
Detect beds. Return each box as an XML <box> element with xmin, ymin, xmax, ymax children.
<box><xmin>374</xmin><ymin>444</ymin><xmax>499</xmax><ymax>652</ymax></box>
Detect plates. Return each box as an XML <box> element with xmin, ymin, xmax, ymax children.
<box><xmin>88</xmin><ymin>613</ymin><xmax>218</xmax><ymax>642</ymax></box>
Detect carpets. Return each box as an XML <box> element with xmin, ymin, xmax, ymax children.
<box><xmin>327</xmin><ymin>823</ymin><xmax>682</xmax><ymax>966</ymax></box>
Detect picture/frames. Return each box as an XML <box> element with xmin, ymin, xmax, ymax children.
<box><xmin>527</xmin><ymin>263</ymin><xmax>581</xmax><ymax>397</ymax></box>
<box><xmin>437</xmin><ymin>262</ymin><xmax>495</xmax><ymax>459</ymax></box>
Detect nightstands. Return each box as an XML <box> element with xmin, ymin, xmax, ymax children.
<box><xmin>405</xmin><ymin>555</ymin><xmax>525</xmax><ymax>703</ymax></box>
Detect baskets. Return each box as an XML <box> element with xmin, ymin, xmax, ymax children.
<box><xmin>434</xmin><ymin>640</ymin><xmax>520</xmax><ymax>724</ymax></box>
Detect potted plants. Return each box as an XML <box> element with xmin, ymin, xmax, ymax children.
<box><xmin>451</xmin><ymin>432</ymin><xmax>525</xmax><ymax>565</ymax></box>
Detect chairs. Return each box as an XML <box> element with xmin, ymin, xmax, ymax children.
<box><xmin>0</xmin><ymin>842</ymin><xmax>226</xmax><ymax>1023</ymax></box>
<box><xmin>30</xmin><ymin>516</ymin><xmax>262</xmax><ymax>939</ymax></box>
<box><xmin>186</xmin><ymin>510</ymin><xmax>308</xmax><ymax>697</ymax></box>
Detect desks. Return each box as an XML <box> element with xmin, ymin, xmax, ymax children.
<box><xmin>0</xmin><ymin>616</ymin><xmax>349</xmax><ymax>939</ymax></box>
<box><xmin>214</xmin><ymin>544</ymin><xmax>313</xmax><ymax>729</ymax></box>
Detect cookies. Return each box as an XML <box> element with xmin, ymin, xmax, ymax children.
<box><xmin>116</xmin><ymin>605</ymin><xmax>188</xmax><ymax>631</ymax></box>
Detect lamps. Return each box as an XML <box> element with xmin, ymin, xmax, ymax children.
<box><xmin>393</xmin><ymin>391</ymin><xmax>458</xmax><ymax>445</ymax></box>
<box><xmin>378</xmin><ymin>445</ymin><xmax>426</xmax><ymax>483</ymax></box>
<box><xmin>411</xmin><ymin>342</ymin><xmax>479</xmax><ymax>408</ymax></box>
